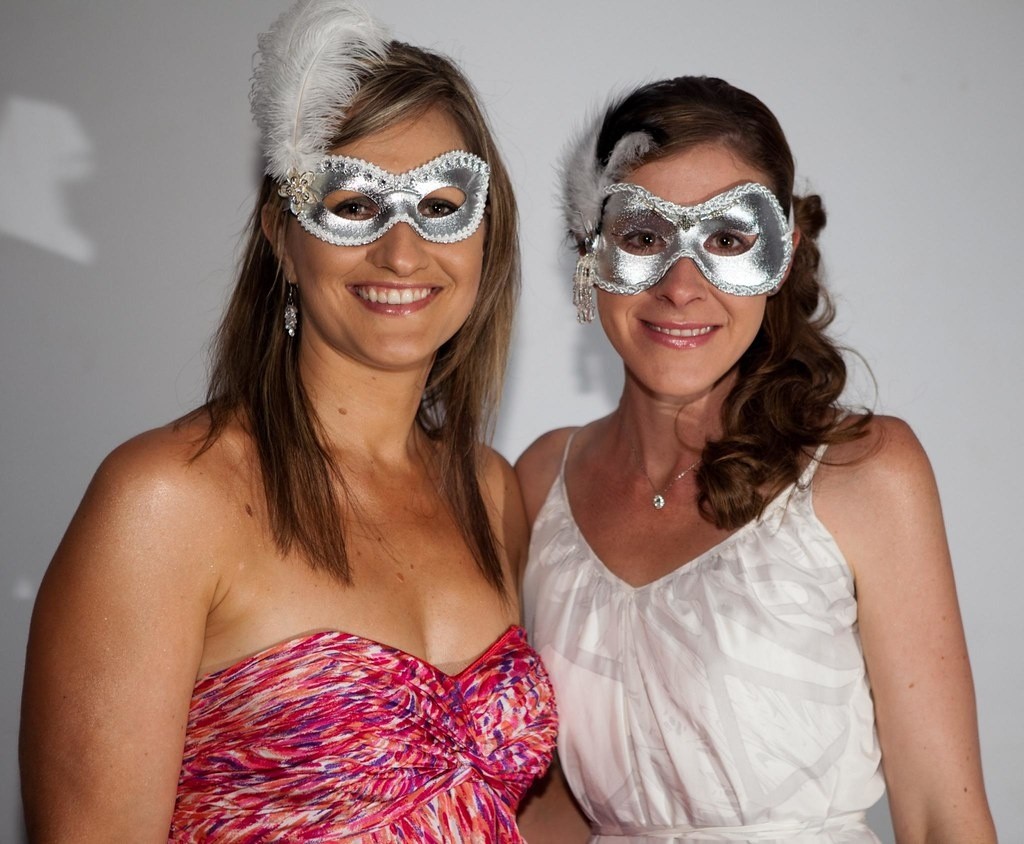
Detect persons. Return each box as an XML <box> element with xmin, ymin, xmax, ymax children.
<box><xmin>508</xmin><ymin>74</ymin><xmax>1000</xmax><ymax>844</ymax></box>
<box><xmin>14</xmin><ymin>37</ymin><xmax>561</xmax><ymax>844</ymax></box>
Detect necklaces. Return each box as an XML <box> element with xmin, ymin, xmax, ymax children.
<box><xmin>618</xmin><ymin>406</ymin><xmax>706</xmax><ymax>512</ymax></box>
